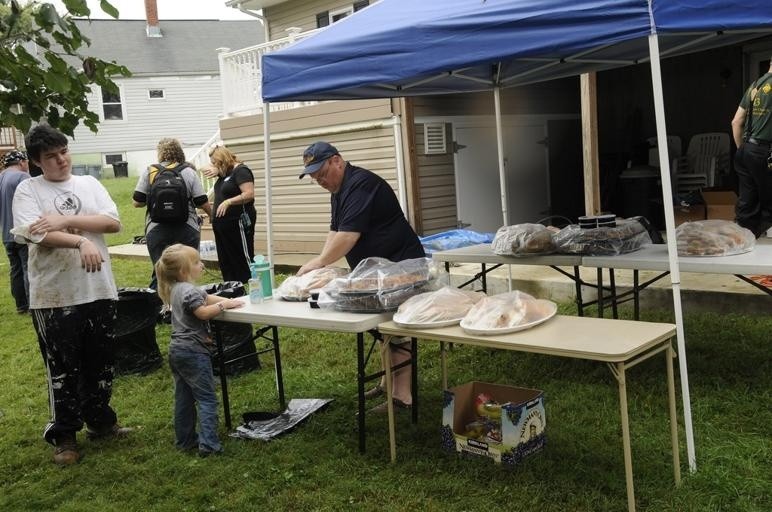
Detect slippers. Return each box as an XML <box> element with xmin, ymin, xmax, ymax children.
<box><xmin>364</xmin><ymin>386</ymin><xmax>386</xmax><ymax>400</ymax></box>
<box><xmin>370</xmin><ymin>398</ymin><xmax>412</xmax><ymax>413</ymax></box>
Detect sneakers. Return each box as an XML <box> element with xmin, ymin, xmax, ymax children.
<box><xmin>54</xmin><ymin>441</ymin><xmax>78</xmax><ymax>464</ymax></box>
<box><xmin>86</xmin><ymin>424</ymin><xmax>133</xmax><ymax>439</ymax></box>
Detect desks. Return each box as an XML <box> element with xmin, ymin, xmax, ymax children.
<box><xmin>581</xmin><ymin>240</ymin><xmax>770</xmax><ymax>319</ymax></box>
<box><xmin>216</xmin><ymin>290</ymin><xmax>420</xmax><ymax>455</ymax></box>
<box><xmin>375</xmin><ymin>312</ymin><xmax>679</xmax><ymax>512</ymax></box>
<box><xmin>424</xmin><ymin>241</ymin><xmax>618</xmax><ymax>347</ymax></box>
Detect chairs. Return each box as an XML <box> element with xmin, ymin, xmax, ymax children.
<box><xmin>620</xmin><ymin>129</ymin><xmax>732</xmax><ymax>195</ymax></box>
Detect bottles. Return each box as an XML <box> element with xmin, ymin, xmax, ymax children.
<box><xmin>199</xmin><ymin>240</ymin><xmax>217</xmax><ymax>259</ymax></box>
<box><xmin>247</xmin><ymin>267</ymin><xmax>264</xmax><ymax>302</ymax></box>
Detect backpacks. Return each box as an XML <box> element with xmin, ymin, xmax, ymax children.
<box><xmin>148</xmin><ymin>162</ymin><xmax>196</xmax><ymax>224</ymax></box>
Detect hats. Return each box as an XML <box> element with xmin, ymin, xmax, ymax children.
<box><xmin>299</xmin><ymin>142</ymin><xmax>337</xmax><ymax>178</ymax></box>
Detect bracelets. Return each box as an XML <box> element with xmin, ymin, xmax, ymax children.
<box><xmin>75</xmin><ymin>235</ymin><xmax>87</xmax><ymax>247</ymax></box>
<box><xmin>216</xmin><ymin>301</ymin><xmax>226</xmax><ymax>311</ymax></box>
<box><xmin>223</xmin><ymin>199</ymin><xmax>232</xmax><ymax>206</ymax></box>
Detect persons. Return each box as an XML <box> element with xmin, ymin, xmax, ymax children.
<box><xmin>730</xmin><ymin>52</ymin><xmax>771</xmax><ymax>238</ymax></box>
<box><xmin>11</xmin><ymin>123</ymin><xmax>138</xmax><ymax>465</ymax></box>
<box><xmin>296</xmin><ymin>140</ymin><xmax>429</xmax><ymax>420</ymax></box>
<box><xmin>1</xmin><ymin>150</ymin><xmax>36</xmax><ymax>314</ymax></box>
<box><xmin>131</xmin><ymin>136</ymin><xmax>212</xmax><ymax>291</ymax></box>
<box><xmin>154</xmin><ymin>242</ymin><xmax>253</xmax><ymax>456</ymax></box>
<box><xmin>202</xmin><ymin>144</ymin><xmax>258</xmax><ymax>285</ymax></box>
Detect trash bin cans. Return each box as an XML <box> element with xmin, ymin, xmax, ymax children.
<box><xmin>112</xmin><ymin>161</ymin><xmax>128</xmax><ymax>177</ymax></box>
<box><xmin>72</xmin><ymin>164</ymin><xmax>86</xmax><ymax>175</ymax></box>
<box><xmin>104</xmin><ymin>286</ymin><xmax>163</xmax><ymax>376</ymax></box>
<box><xmin>200</xmin><ymin>281</ymin><xmax>261</xmax><ymax>376</ymax></box>
<box><xmin>87</xmin><ymin>165</ymin><xmax>101</xmax><ymax>180</ymax></box>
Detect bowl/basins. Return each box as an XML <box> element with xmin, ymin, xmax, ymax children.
<box><xmin>578</xmin><ymin>215</ymin><xmax>616</xmax><ymax>230</ymax></box>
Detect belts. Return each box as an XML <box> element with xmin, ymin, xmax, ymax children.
<box><xmin>744</xmin><ymin>138</ymin><xmax>771</xmax><ymax>145</ymax></box>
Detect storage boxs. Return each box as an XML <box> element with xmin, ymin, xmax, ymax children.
<box><xmin>438</xmin><ymin>377</ymin><xmax>551</xmax><ymax>460</ymax></box>
<box><xmin>697</xmin><ymin>183</ymin><xmax>738</xmax><ymax>219</ymax></box>
<box><xmin>674</xmin><ymin>202</ymin><xmax>706</xmax><ymax>228</ymax></box>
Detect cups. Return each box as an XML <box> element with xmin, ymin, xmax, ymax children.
<box><xmin>249</xmin><ymin>261</ymin><xmax>274</xmax><ymax>302</ymax></box>
